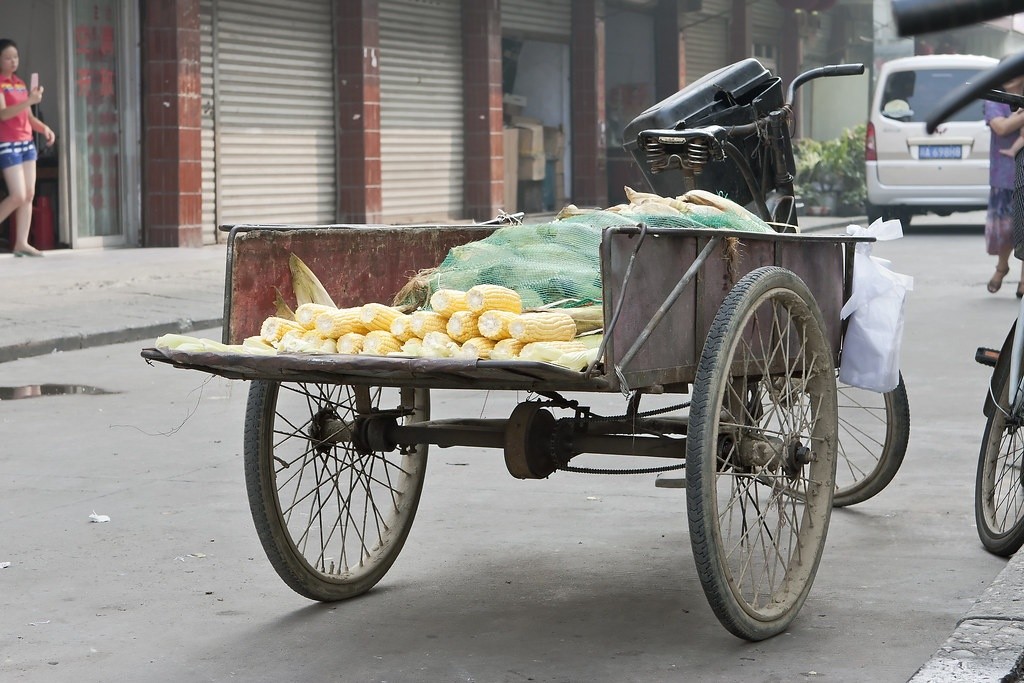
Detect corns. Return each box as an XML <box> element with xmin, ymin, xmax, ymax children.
<box><xmin>611</xmin><ymin>186</ymin><xmax>752</xmax><ymax>219</ymax></box>
<box><xmin>242</xmin><ymin>283</ymin><xmax>591</xmax><ymax>363</ymax></box>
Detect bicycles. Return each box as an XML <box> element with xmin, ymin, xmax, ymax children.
<box><xmin>891</xmin><ymin>0</ymin><xmax>1024</xmax><ymax>557</ymax></box>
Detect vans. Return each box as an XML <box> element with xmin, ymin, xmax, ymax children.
<box><xmin>866</xmin><ymin>53</ymin><xmax>1002</xmax><ymax>229</ymax></box>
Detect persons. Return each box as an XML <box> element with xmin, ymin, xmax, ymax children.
<box><xmin>982</xmin><ymin>54</ymin><xmax>1024</xmax><ymax>298</ymax></box>
<box><xmin>0</xmin><ymin>39</ymin><xmax>55</xmax><ymax>258</ymax></box>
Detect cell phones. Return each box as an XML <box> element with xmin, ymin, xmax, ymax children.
<box><xmin>30</xmin><ymin>72</ymin><xmax>39</xmax><ymax>91</ymax></box>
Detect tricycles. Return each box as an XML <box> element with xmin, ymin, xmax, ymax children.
<box><xmin>141</xmin><ymin>57</ymin><xmax>911</xmax><ymax>644</ymax></box>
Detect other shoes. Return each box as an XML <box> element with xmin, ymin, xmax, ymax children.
<box><xmin>14</xmin><ymin>246</ymin><xmax>44</xmax><ymax>257</ymax></box>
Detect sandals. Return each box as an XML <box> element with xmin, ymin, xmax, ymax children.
<box><xmin>987</xmin><ymin>266</ymin><xmax>1009</xmax><ymax>293</ymax></box>
<box><xmin>1016</xmin><ymin>282</ymin><xmax>1024</xmax><ymax>298</ymax></box>
